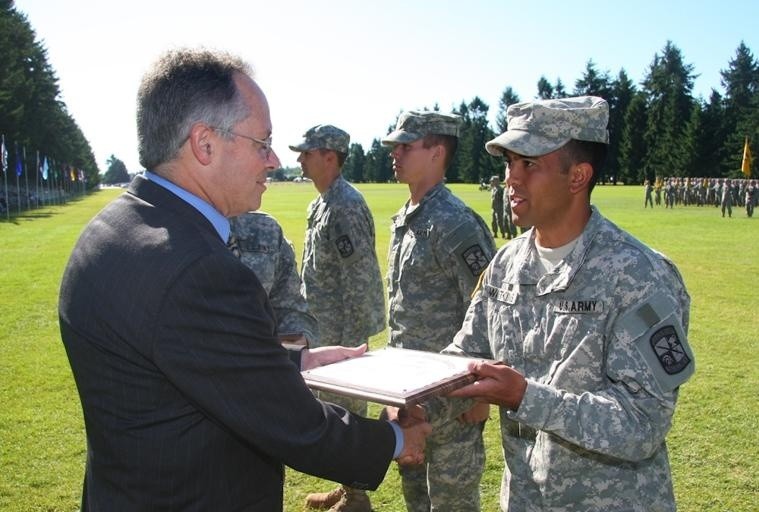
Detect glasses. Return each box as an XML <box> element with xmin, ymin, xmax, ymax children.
<box><xmin>178</xmin><ymin>126</ymin><xmax>273</xmax><ymax>155</ymax></box>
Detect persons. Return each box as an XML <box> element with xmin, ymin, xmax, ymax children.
<box><xmin>477</xmin><ymin>174</ymin><xmax>533</xmax><ymax>240</ymax></box>
<box><xmin>644</xmin><ymin>169</ymin><xmax>759</xmax><ymax>218</ymax></box>
<box><xmin>381</xmin><ymin>108</ymin><xmax>495</xmax><ymax>512</ymax></box>
<box><xmin>223</xmin><ymin>213</ymin><xmax>316</xmax><ymax>356</ymax></box>
<box><xmin>391</xmin><ymin>95</ymin><xmax>698</xmax><ymax>512</ymax></box>
<box><xmin>287</xmin><ymin>123</ymin><xmax>388</xmax><ymax>512</ymax></box>
<box><xmin>58</xmin><ymin>48</ymin><xmax>433</xmax><ymax>511</ymax></box>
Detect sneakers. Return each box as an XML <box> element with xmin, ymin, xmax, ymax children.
<box><xmin>307</xmin><ymin>488</ymin><xmax>345</xmax><ymax>507</ymax></box>
<box><xmin>328</xmin><ymin>494</ymin><xmax>370</xmax><ymax>512</ymax></box>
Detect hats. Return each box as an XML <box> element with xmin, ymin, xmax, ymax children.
<box><xmin>382</xmin><ymin>112</ymin><xmax>465</xmax><ymax>145</ymax></box>
<box><xmin>483</xmin><ymin>95</ymin><xmax>611</xmax><ymax>157</ymax></box>
<box><xmin>289</xmin><ymin>124</ymin><xmax>350</xmax><ymax>155</ymax></box>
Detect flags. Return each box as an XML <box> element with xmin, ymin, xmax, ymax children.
<box><xmin>0</xmin><ymin>141</ymin><xmax>92</xmax><ymax>183</ymax></box>
<box><xmin>740</xmin><ymin>134</ymin><xmax>754</xmax><ymax>178</ymax></box>
